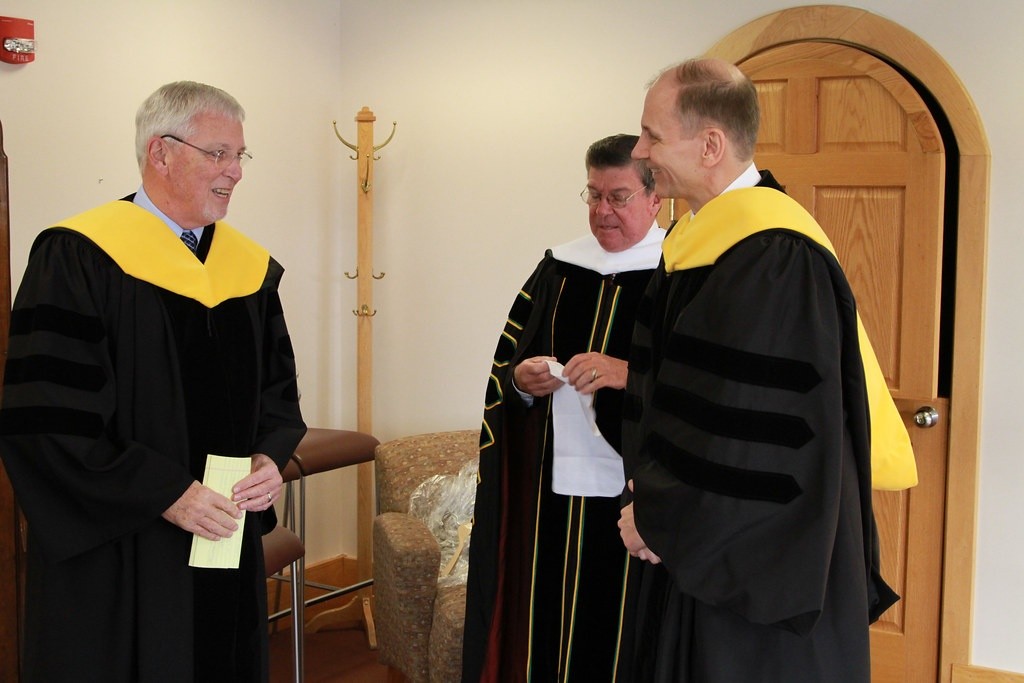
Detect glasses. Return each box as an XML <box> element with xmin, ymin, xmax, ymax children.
<box><xmin>580</xmin><ymin>182</ymin><xmax>647</xmax><ymax>209</ymax></box>
<box><xmin>162</xmin><ymin>134</ymin><xmax>253</xmax><ymax>169</ymax></box>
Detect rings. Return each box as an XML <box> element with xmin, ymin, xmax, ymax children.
<box><xmin>267</xmin><ymin>492</ymin><xmax>272</xmax><ymax>503</ymax></box>
<box><xmin>591</xmin><ymin>369</ymin><xmax>597</xmax><ymax>379</ymax></box>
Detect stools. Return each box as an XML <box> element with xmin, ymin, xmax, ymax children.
<box><xmin>263</xmin><ymin>428</ymin><xmax>381</xmax><ymax>683</ymax></box>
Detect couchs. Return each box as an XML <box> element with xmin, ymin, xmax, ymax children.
<box><xmin>372</xmin><ymin>430</ymin><xmax>481</xmax><ymax>683</ymax></box>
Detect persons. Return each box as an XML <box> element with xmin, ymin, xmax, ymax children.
<box><xmin>0</xmin><ymin>81</ymin><xmax>308</xmax><ymax>683</ymax></box>
<box><xmin>618</xmin><ymin>59</ymin><xmax>918</xmax><ymax>683</ymax></box>
<box><xmin>460</xmin><ymin>134</ymin><xmax>666</xmax><ymax>683</ymax></box>
